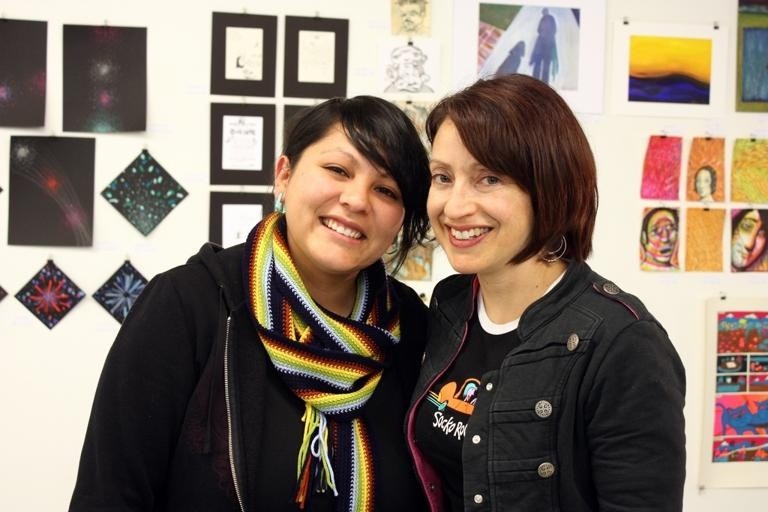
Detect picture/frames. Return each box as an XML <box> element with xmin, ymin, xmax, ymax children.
<box><xmin>700</xmin><ymin>294</ymin><xmax>768</xmax><ymax>491</ymax></box>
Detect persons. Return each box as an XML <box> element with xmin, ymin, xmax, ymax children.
<box><xmin>690</xmin><ymin>166</ymin><xmax>717</xmax><ymax>204</ymax></box>
<box><xmin>405</xmin><ymin>72</ymin><xmax>685</xmax><ymax>511</ymax></box>
<box><xmin>69</xmin><ymin>95</ymin><xmax>430</xmax><ymax>512</ymax></box>
<box><xmin>638</xmin><ymin>207</ymin><xmax>679</xmax><ymax>272</ymax></box>
<box><xmin>731</xmin><ymin>208</ymin><xmax>767</xmax><ymax>272</ymax></box>
<box><xmin>396</xmin><ymin>0</ymin><xmax>428</xmax><ymax>34</ymax></box>
